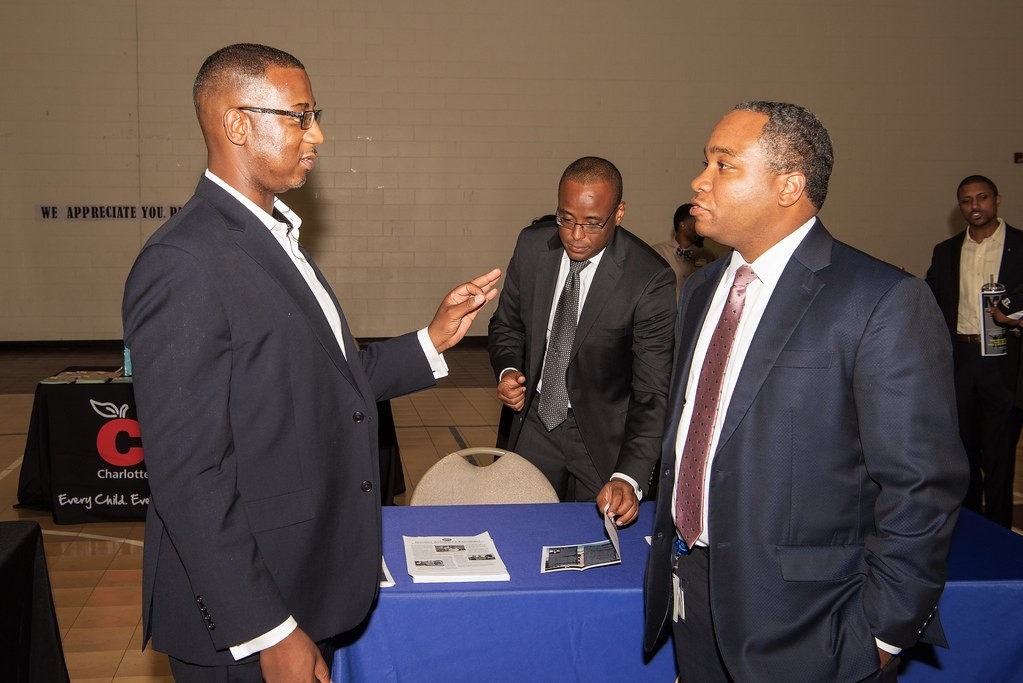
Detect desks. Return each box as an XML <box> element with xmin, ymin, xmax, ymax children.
<box><xmin>330</xmin><ymin>502</ymin><xmax>1023</xmax><ymax>683</ymax></box>
<box><xmin>12</xmin><ymin>366</ymin><xmax>405</xmax><ymax>525</ymax></box>
<box><xmin>0</xmin><ymin>521</ymin><xmax>71</xmax><ymax>682</ymax></box>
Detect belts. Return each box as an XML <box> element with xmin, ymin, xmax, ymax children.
<box><xmin>956</xmin><ymin>334</ymin><xmax>981</xmax><ymax>344</ymax></box>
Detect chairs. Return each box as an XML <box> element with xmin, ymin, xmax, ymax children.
<box><xmin>409</xmin><ymin>446</ymin><xmax>561</xmax><ymax>506</ymax></box>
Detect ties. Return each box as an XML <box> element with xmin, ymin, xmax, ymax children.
<box><xmin>676</xmin><ymin>265</ymin><xmax>758</xmax><ymax>549</ymax></box>
<box><xmin>536</xmin><ymin>258</ymin><xmax>590</xmax><ymax>431</ymax></box>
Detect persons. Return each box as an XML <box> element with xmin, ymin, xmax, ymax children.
<box><xmin>667</xmin><ymin>102</ymin><xmax>969</xmax><ymax>683</ymax></box>
<box><xmin>650</xmin><ymin>203</ymin><xmax>718</xmax><ymax>302</ymax></box>
<box><xmin>120</xmin><ymin>44</ymin><xmax>502</xmax><ymax>683</ymax></box>
<box><xmin>488</xmin><ymin>156</ymin><xmax>678</xmax><ymax>528</ymax></box>
<box><xmin>924</xmin><ymin>176</ymin><xmax>1023</xmax><ymax>530</ymax></box>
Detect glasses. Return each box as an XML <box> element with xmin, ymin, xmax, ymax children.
<box><xmin>555</xmin><ymin>206</ymin><xmax>617</xmax><ymax>233</ymax></box>
<box><xmin>222</xmin><ymin>107</ymin><xmax>323</xmax><ymax>130</ymax></box>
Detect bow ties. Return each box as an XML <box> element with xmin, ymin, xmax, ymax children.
<box><xmin>676</xmin><ymin>247</ymin><xmax>694</xmax><ymax>261</ymax></box>
